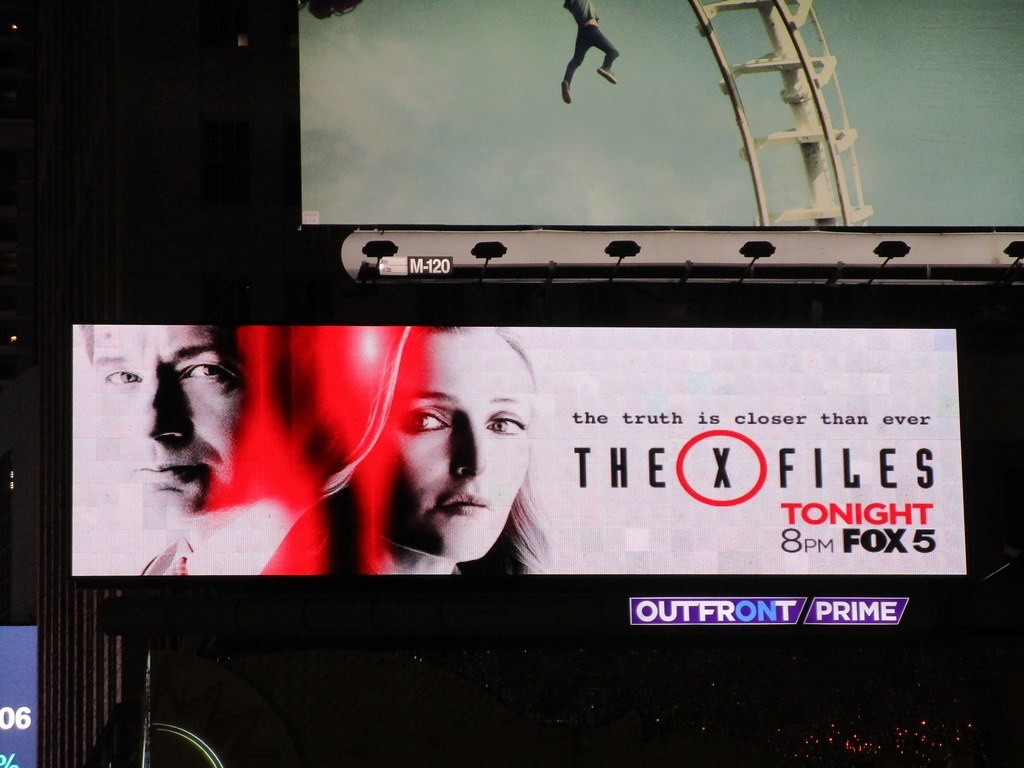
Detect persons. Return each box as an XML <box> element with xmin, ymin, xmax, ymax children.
<box><xmin>367</xmin><ymin>326</ymin><xmax>553</xmax><ymax>575</ymax></box>
<box><xmin>80</xmin><ymin>327</ymin><xmax>332</xmax><ymax>575</ymax></box>
<box><xmin>561</xmin><ymin>0</ymin><xmax>619</xmax><ymax>104</ymax></box>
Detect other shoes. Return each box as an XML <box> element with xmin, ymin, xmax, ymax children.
<box><xmin>561</xmin><ymin>80</ymin><xmax>571</xmax><ymax>104</ymax></box>
<box><xmin>596</xmin><ymin>66</ymin><xmax>618</xmax><ymax>85</ymax></box>
